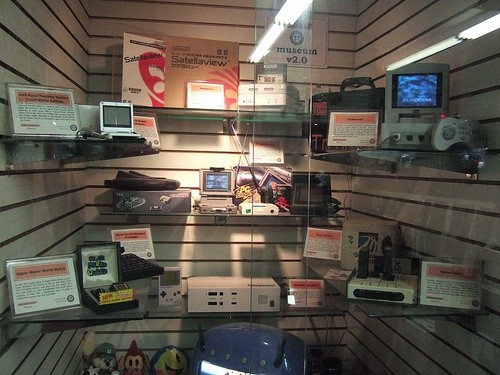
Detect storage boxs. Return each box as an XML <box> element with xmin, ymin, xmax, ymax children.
<box><xmin>340</xmin><ymin>217</ymin><xmax>398</xmax><ymax>275</ymax></box>
<box><xmin>237</xmin><ymin>84</ymin><xmax>299</xmax><ymax>114</ymax></box>
<box><xmin>188</xmin><ymin>276</ymin><xmax>281</xmax><ymax>313</ymax></box>
<box><xmin>113</xmin><ymin>189</ymin><xmax>191</xmax><ymax>213</ymax></box>
<box><xmin>75</xmin><ymin>242</ymin><xmax>139</xmax><ymax>316</ymax></box>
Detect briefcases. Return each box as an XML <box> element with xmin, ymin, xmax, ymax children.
<box><xmin>310</xmin><ymin>75</ymin><xmax>389</xmax><ymax>152</ymax></box>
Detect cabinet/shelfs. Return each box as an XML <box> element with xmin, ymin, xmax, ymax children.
<box><xmin>0</xmin><ymin>0</ymin><xmax>500</xmax><ymax>375</ymax></box>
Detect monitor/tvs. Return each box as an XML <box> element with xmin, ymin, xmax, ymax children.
<box><xmin>100</xmin><ymin>102</ymin><xmax>133</xmax><ymax>133</ymax></box>
<box><xmin>199</xmin><ymin>168</ymin><xmax>235</xmax><ymax>195</ymax></box>
<box><xmin>161</xmin><ymin>267</ymin><xmax>182</xmax><ymax>288</ymax></box>
<box><xmin>385</xmin><ymin>63</ymin><xmax>449</xmax><ymax>123</ymax></box>
<box><xmin>292</xmin><ymin>184</ymin><xmax>329</xmax><ymax>215</ymax></box>
